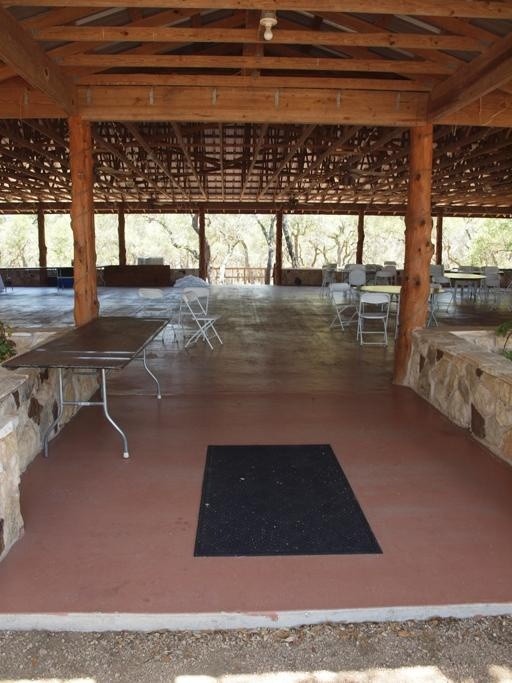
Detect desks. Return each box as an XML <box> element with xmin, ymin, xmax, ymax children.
<box><xmin>0</xmin><ymin>315</ymin><xmax>172</xmax><ymax>459</ymax></box>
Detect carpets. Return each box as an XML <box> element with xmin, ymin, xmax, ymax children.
<box><xmin>193</xmin><ymin>443</ymin><xmax>388</xmax><ymax>556</ymax></box>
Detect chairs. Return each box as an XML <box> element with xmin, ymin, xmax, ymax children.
<box><xmin>137</xmin><ymin>286</ymin><xmax>223</xmax><ymax>352</ymax></box>
<box><xmin>320</xmin><ymin>260</ymin><xmax>503</xmax><ymax>347</ymax></box>
